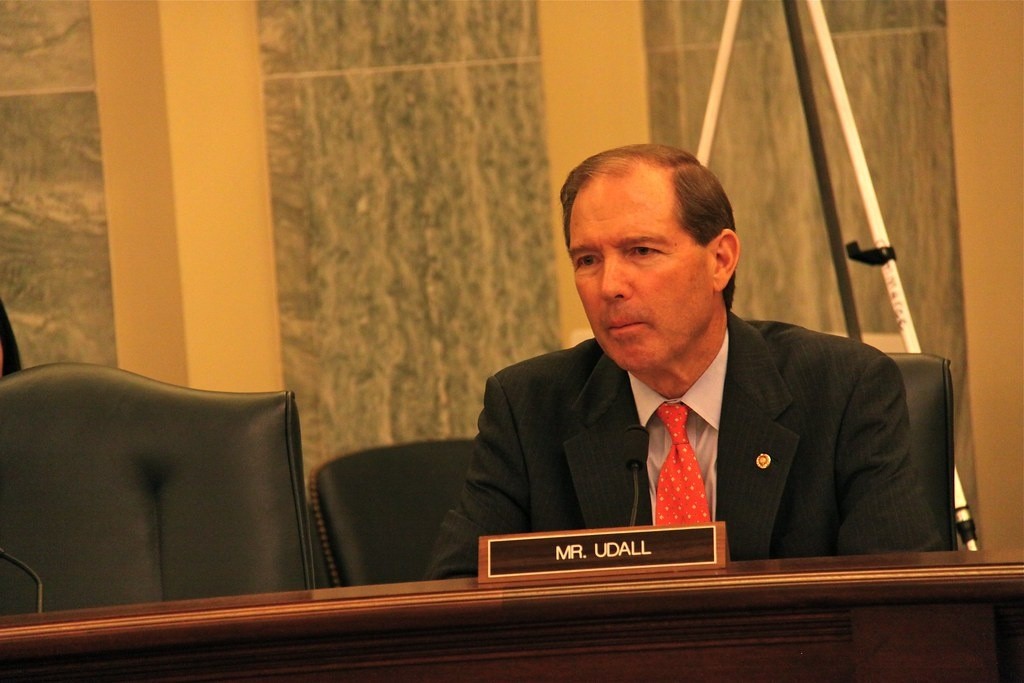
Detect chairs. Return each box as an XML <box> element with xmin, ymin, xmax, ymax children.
<box><xmin>0</xmin><ymin>361</ymin><xmax>313</xmax><ymax>616</ymax></box>
<box><xmin>313</xmin><ymin>435</ymin><xmax>478</xmax><ymax>586</ymax></box>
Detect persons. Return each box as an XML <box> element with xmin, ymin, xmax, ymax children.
<box><xmin>418</xmin><ymin>144</ymin><xmax>948</xmax><ymax>583</ymax></box>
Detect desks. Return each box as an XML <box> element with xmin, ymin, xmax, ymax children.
<box><xmin>1</xmin><ymin>547</ymin><xmax>1023</xmax><ymax>683</ymax></box>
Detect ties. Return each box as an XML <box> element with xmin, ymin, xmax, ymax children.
<box><xmin>653</xmin><ymin>403</ymin><xmax>713</xmax><ymax>524</ymax></box>
